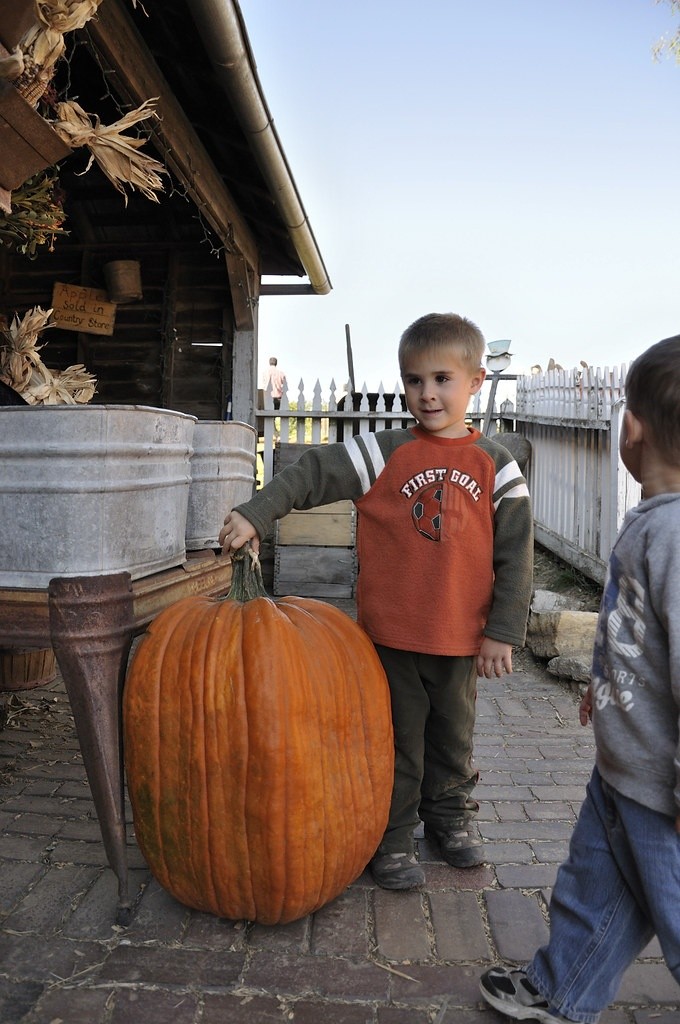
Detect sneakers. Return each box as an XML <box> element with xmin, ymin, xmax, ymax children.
<box><xmin>479</xmin><ymin>966</ymin><xmax>584</xmax><ymax>1024</ymax></box>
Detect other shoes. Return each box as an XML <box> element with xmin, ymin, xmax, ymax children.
<box><xmin>424</xmin><ymin>822</ymin><xmax>485</xmax><ymax>867</ymax></box>
<box><xmin>370</xmin><ymin>852</ymin><xmax>425</xmax><ymax>889</ymax></box>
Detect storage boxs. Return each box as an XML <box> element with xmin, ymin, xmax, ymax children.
<box><xmin>0</xmin><ymin>405</ymin><xmax>198</xmax><ymax>589</ymax></box>
<box><xmin>272</xmin><ymin>441</ymin><xmax>357</xmax><ymax>600</ymax></box>
<box><xmin>181</xmin><ymin>420</ymin><xmax>259</xmax><ymax>551</ymax></box>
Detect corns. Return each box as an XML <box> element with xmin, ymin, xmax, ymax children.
<box><xmin>9</xmin><ymin>58</ymin><xmax>58</xmax><ymax>108</ymax></box>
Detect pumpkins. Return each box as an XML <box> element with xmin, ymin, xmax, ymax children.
<box><xmin>123</xmin><ymin>541</ymin><xmax>395</xmax><ymax>927</ymax></box>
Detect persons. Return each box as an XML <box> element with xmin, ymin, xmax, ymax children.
<box><xmin>482</xmin><ymin>336</ymin><xmax>680</xmax><ymax>1024</ymax></box>
<box><xmin>219</xmin><ymin>311</ymin><xmax>535</xmax><ymax>887</ymax></box>
<box><xmin>262</xmin><ymin>357</ymin><xmax>285</xmax><ymax>409</ymax></box>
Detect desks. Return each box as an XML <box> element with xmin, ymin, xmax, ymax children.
<box><xmin>2</xmin><ymin>547</ymin><xmax>244</xmax><ymax>913</ymax></box>
